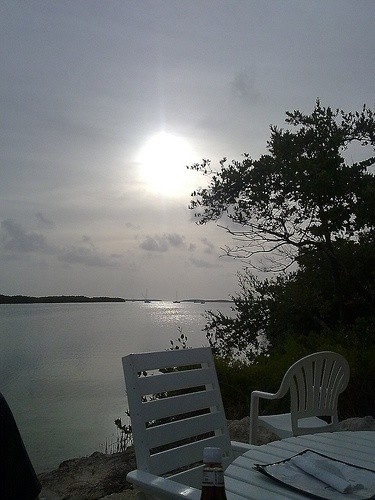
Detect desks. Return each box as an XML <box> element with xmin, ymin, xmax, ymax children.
<box><xmin>222</xmin><ymin>430</ymin><xmax>374</xmax><ymax>499</ymax></box>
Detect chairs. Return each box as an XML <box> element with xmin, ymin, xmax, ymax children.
<box><xmin>121</xmin><ymin>347</ymin><xmax>258</xmax><ymax>500</ymax></box>
<box><xmin>249</xmin><ymin>350</ymin><xmax>350</xmax><ymax>447</ymax></box>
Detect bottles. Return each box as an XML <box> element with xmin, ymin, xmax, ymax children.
<box><xmin>199</xmin><ymin>447</ymin><xmax>227</xmax><ymax>500</ymax></box>
